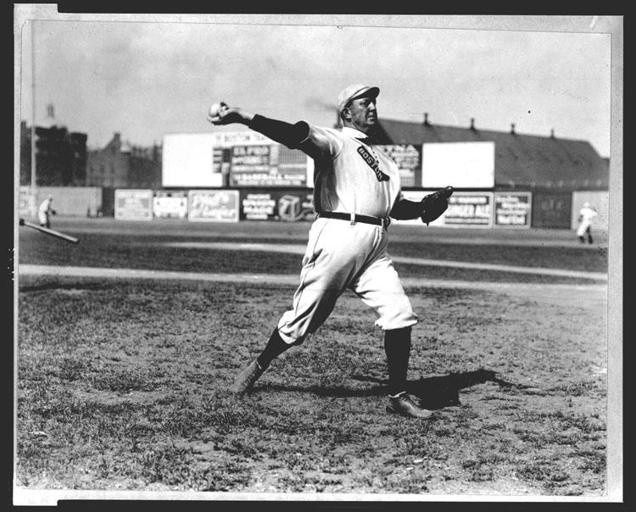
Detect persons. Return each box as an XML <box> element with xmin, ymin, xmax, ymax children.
<box><xmin>575</xmin><ymin>203</ymin><xmax>599</xmax><ymax>244</ymax></box>
<box><xmin>206</xmin><ymin>83</ymin><xmax>452</xmax><ymax>424</ymax></box>
<box><xmin>37</xmin><ymin>194</ymin><xmax>56</xmax><ymax>229</ymax></box>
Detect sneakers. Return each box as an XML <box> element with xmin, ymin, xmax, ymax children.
<box><xmin>386</xmin><ymin>392</ymin><xmax>433</xmax><ymax>420</ymax></box>
<box><xmin>231</xmin><ymin>359</ymin><xmax>265</xmax><ymax>395</ymax></box>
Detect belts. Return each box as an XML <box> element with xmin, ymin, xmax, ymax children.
<box><xmin>318</xmin><ymin>212</ymin><xmax>380</xmax><ymax>226</ymax></box>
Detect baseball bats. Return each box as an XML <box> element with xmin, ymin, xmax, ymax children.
<box><xmin>23</xmin><ymin>220</ymin><xmax>79</xmax><ymax>245</ymax></box>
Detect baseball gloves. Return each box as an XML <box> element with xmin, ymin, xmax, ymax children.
<box><xmin>420</xmin><ymin>187</ymin><xmax>453</xmax><ymax>223</ymax></box>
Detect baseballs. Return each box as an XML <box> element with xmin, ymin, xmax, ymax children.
<box><xmin>209</xmin><ymin>101</ymin><xmax>227</xmax><ymax>117</ymax></box>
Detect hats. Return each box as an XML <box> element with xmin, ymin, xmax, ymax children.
<box><xmin>336</xmin><ymin>84</ymin><xmax>379</xmax><ymax>112</ymax></box>
